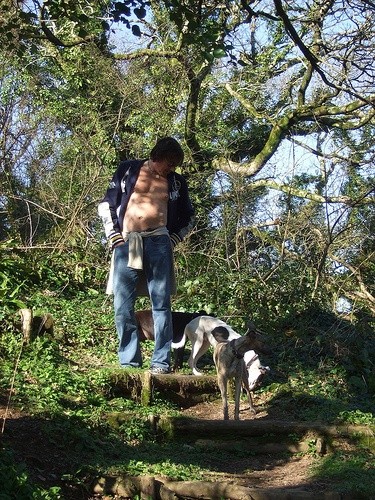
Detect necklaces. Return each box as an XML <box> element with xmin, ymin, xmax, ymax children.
<box><xmin>147</xmin><ymin>161</ymin><xmax>160</xmax><ymax>179</ymax></box>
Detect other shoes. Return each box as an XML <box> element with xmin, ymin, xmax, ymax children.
<box><xmin>150</xmin><ymin>364</ymin><xmax>168</xmax><ymax>375</ymax></box>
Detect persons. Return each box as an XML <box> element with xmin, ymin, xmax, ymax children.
<box><xmin>98</xmin><ymin>137</ymin><xmax>196</xmax><ymax>373</ymax></box>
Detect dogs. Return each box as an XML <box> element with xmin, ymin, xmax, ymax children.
<box><xmin>211</xmin><ymin>326</ymin><xmax>265</xmax><ymax>422</ymax></box>
<box><xmin>133</xmin><ymin>310</ymin><xmax>216</xmax><ymax>368</ymax></box>
<box><xmin>171</xmin><ymin>316</ymin><xmax>271</xmax><ymax>391</ymax></box>
